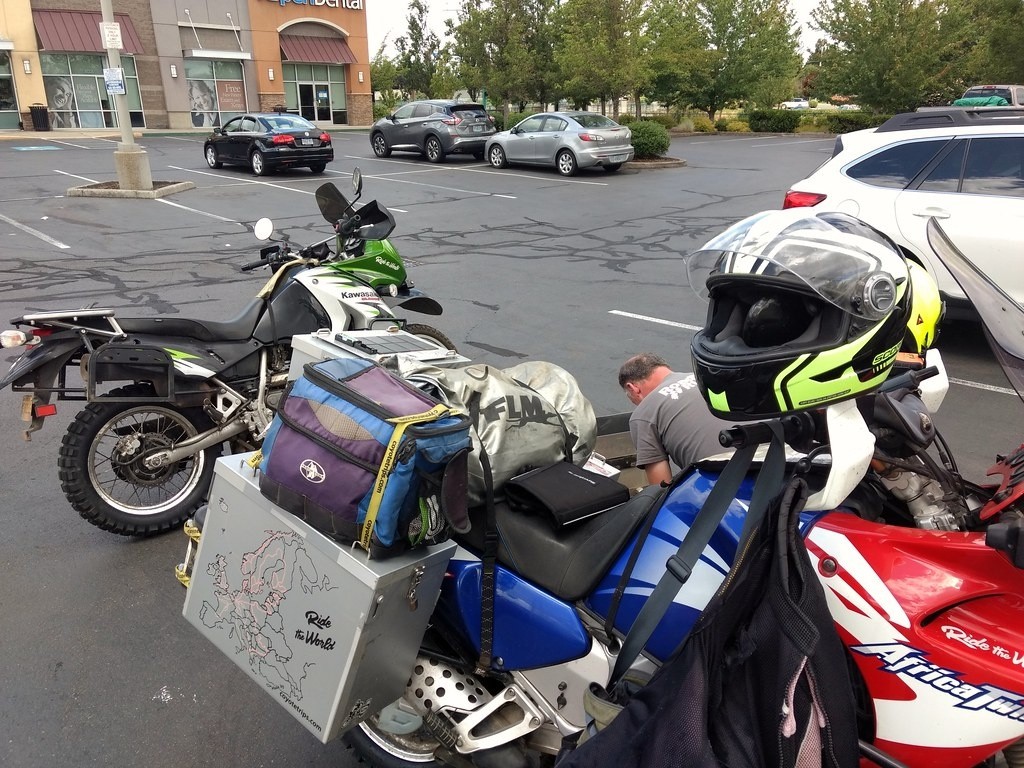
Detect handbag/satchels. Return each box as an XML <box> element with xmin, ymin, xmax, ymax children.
<box><xmin>251</xmin><ymin>356</ymin><xmax>472</xmax><ymax>559</ymax></box>
<box><xmin>553</xmin><ymin>420</ymin><xmax>876</xmax><ymax>768</ymax></box>
<box><xmin>379</xmin><ymin>353</ymin><xmax>599</xmax><ymax>494</ymax></box>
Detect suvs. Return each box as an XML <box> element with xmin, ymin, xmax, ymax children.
<box><xmin>369</xmin><ymin>99</ymin><xmax>497</xmax><ymax>163</ymax></box>
<box><xmin>779</xmin><ymin>103</ymin><xmax>1024</xmax><ymax>335</ymax></box>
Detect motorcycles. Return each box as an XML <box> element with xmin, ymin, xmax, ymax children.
<box><xmin>0</xmin><ymin>167</ymin><xmax>460</xmax><ymax>535</ymax></box>
<box><xmin>172</xmin><ymin>295</ymin><xmax>1024</xmax><ymax>768</ymax></box>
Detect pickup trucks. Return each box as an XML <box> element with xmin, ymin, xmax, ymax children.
<box><xmin>779</xmin><ymin>98</ymin><xmax>810</xmax><ymax>110</ymax></box>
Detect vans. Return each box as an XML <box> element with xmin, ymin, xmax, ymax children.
<box><xmin>961</xmin><ymin>84</ymin><xmax>1024</xmax><ymax>110</ymax></box>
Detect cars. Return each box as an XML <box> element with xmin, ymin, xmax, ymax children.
<box><xmin>203</xmin><ymin>112</ymin><xmax>335</xmax><ymax>176</ymax></box>
<box><xmin>485</xmin><ymin>110</ymin><xmax>634</xmax><ymax>178</ymax></box>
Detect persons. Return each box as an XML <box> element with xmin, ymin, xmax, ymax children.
<box><xmin>187</xmin><ymin>79</ymin><xmax>220</xmax><ymax>128</ymax></box>
<box><xmin>618</xmin><ymin>352</ymin><xmax>780</xmax><ymax>488</ymax></box>
<box><xmin>44</xmin><ymin>76</ymin><xmax>76</xmax><ymax>128</ymax></box>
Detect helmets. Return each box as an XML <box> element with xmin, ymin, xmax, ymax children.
<box><xmin>685</xmin><ymin>206</ymin><xmax>912</xmax><ymax>419</ymax></box>
<box><xmin>896</xmin><ymin>241</ymin><xmax>945</xmax><ymax>367</ymax></box>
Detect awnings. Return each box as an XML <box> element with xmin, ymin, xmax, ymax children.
<box><xmin>279</xmin><ymin>34</ymin><xmax>358</xmax><ymax>65</ymax></box>
<box><xmin>32</xmin><ymin>8</ymin><xmax>145</xmax><ymax>55</ymax></box>
